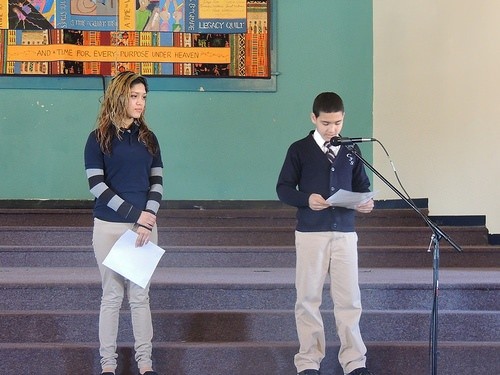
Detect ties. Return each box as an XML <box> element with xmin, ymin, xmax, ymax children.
<box><xmin>323</xmin><ymin>141</ymin><xmax>335</xmax><ymax>163</ymax></box>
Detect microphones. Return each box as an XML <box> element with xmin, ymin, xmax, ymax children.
<box><xmin>330</xmin><ymin>136</ymin><xmax>376</xmax><ymax>146</ymax></box>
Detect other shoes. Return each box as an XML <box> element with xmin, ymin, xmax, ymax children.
<box><xmin>138</xmin><ymin>366</ymin><xmax>158</xmax><ymax>375</ymax></box>
<box><xmin>344</xmin><ymin>367</ymin><xmax>375</xmax><ymax>375</ymax></box>
<box><xmin>101</xmin><ymin>371</ymin><xmax>116</xmax><ymax>375</ymax></box>
<box><xmin>298</xmin><ymin>369</ymin><xmax>320</xmax><ymax>375</ymax></box>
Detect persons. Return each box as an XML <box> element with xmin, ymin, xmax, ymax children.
<box><xmin>84</xmin><ymin>71</ymin><xmax>164</xmax><ymax>375</ymax></box>
<box><xmin>276</xmin><ymin>92</ymin><xmax>374</xmax><ymax>375</ymax></box>
